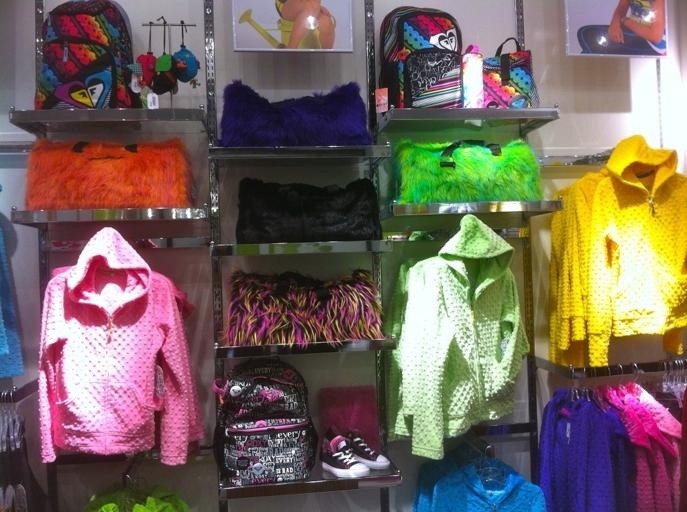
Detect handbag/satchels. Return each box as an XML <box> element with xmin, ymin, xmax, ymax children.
<box><xmin>227</xmin><ymin>270</ymin><xmax>383</xmax><ymax>346</ymax></box>
<box><xmin>236</xmin><ymin>178</ymin><xmax>382</xmax><ymax>244</ymax></box>
<box><xmin>392</xmin><ymin>138</ymin><xmax>540</xmax><ymax>203</ymax></box>
<box><xmin>482</xmin><ymin>37</ymin><xmax>540</xmax><ymax>109</ymax></box>
<box><xmin>25</xmin><ymin>139</ymin><xmax>191</xmax><ymax>211</ymax></box>
<box><xmin>221</xmin><ymin>80</ymin><xmax>370</xmax><ymax>148</ymax></box>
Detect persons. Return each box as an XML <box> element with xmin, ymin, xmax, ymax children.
<box><xmin>274</xmin><ymin>0</ymin><xmax>336</xmax><ymax>50</ymax></box>
<box><xmin>574</xmin><ymin>0</ymin><xmax>665</xmax><ymax>55</ymax></box>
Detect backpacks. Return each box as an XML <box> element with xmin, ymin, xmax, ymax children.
<box><xmin>379</xmin><ymin>6</ymin><xmax>462</xmax><ymax>109</ymax></box>
<box><xmin>214</xmin><ymin>358</ymin><xmax>318</xmax><ymax>487</ymax></box>
<box><xmin>34</xmin><ymin>0</ymin><xmax>141</xmax><ymax>109</ymax></box>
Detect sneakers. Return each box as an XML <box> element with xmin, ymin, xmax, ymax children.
<box><xmin>320</xmin><ymin>433</ymin><xmax>370</xmax><ymax>478</ymax></box>
<box><xmin>344</xmin><ymin>430</ymin><xmax>390</xmax><ymax>470</ymax></box>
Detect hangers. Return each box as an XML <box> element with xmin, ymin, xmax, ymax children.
<box><xmin>565</xmin><ymin>357</ymin><xmax>686</xmax><ymax>411</ymax></box>
<box><xmin>462</xmin><ymin>433</ymin><xmax>508</xmax><ymax>491</ymax></box>
<box><xmin>92</xmin><ymin>457</ymin><xmax>158</xmax><ymax>510</ymax></box>
<box><xmin>1</xmin><ymin>381</ymin><xmax>26</xmax><ymax>501</ymax></box>
